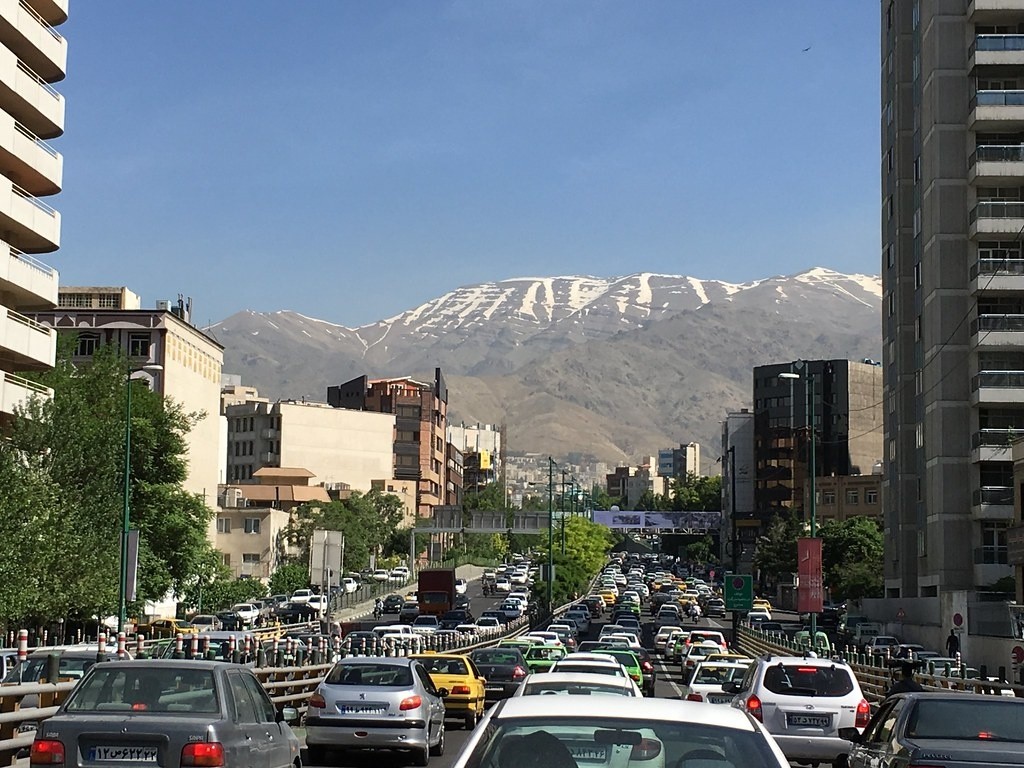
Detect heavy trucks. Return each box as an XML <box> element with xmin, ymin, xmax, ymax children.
<box><xmin>417</xmin><ymin>567</ymin><xmax>456</xmax><ymax>615</ymax></box>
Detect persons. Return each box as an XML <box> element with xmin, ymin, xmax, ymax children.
<box><xmin>686</xmin><ymin>597</ymin><xmax>701</xmax><ymax>618</ymax></box>
<box><xmin>946</xmin><ymin>629</ymin><xmax>958</xmax><ymax>657</ymax></box>
<box><xmin>132</xmin><ymin>675</ymin><xmax>167</xmax><ymax>711</ymax></box>
<box><xmin>887</xmin><ymin>664</ymin><xmax>922</xmax><ymax>696</ymax></box>
<box><xmin>372</xmin><ymin>598</ymin><xmax>383</xmax><ymax>619</ymax></box>
<box><xmin>330</xmin><ymin>616</ymin><xmax>343</xmax><ymax>638</ymax></box>
<box><xmin>482</xmin><ymin>580</ymin><xmax>497</xmax><ymax>591</ymax></box>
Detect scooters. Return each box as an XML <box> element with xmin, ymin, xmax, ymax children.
<box><xmin>685</xmin><ymin>606</ymin><xmax>692</xmax><ymax>618</ymax></box>
<box><xmin>692</xmin><ymin>609</ymin><xmax>701</xmax><ymax>625</ymax></box>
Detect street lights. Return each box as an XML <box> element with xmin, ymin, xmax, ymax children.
<box><xmin>557</xmin><ymin>492</ymin><xmax>593</xmax><ymax>520</ymax></box>
<box><xmin>118</xmin><ymin>356</ymin><xmax>163</xmax><ymax>629</ymax></box>
<box><xmin>529</xmin><ymin>481</ymin><xmax>572</xmax><ymax>613</ymax></box>
<box><xmin>779</xmin><ymin>371</ymin><xmax>817</xmax><ymax>651</ymax></box>
<box><xmin>544</xmin><ymin>488</ymin><xmax>582</xmax><ymax>554</ymax></box>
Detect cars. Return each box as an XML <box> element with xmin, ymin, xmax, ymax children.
<box><xmin>742</xmin><ymin>599</ymin><xmax>1015</xmax><ymax>696</ymax></box>
<box><xmin>720</xmin><ymin>649</ymin><xmax>870</xmax><ymax>763</ymax></box>
<box><xmin>451</xmin><ymin>696</ymin><xmax>791</xmax><ymax>768</ymax></box>
<box><xmin>138</xmin><ymin>566</ymin><xmax>410</xmax><ymax>637</ymax></box>
<box><xmin>304</xmin><ymin>654</ymin><xmax>448</xmax><ymax>764</ymax></box>
<box><xmin>471</xmin><ymin>550</ymin><xmax>754</xmax><ymax>705</ymax></box>
<box><xmin>31</xmin><ymin>660</ymin><xmax>302</xmax><ymax>768</ymax></box>
<box><xmin>409</xmin><ymin>648</ymin><xmax>485</xmax><ymax>728</ymax></box>
<box><xmin>482</xmin><ymin>549</ymin><xmax>546</xmax><ymax>593</ymax></box>
<box><xmin>837</xmin><ymin>688</ymin><xmax>1024</xmax><ymax>766</ymax></box>
<box><xmin>1</xmin><ymin>579</ymin><xmax>531</xmax><ymax>685</ymax></box>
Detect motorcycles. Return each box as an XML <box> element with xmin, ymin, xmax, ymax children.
<box><xmin>374</xmin><ymin>604</ymin><xmax>383</xmax><ymax>620</ymax></box>
